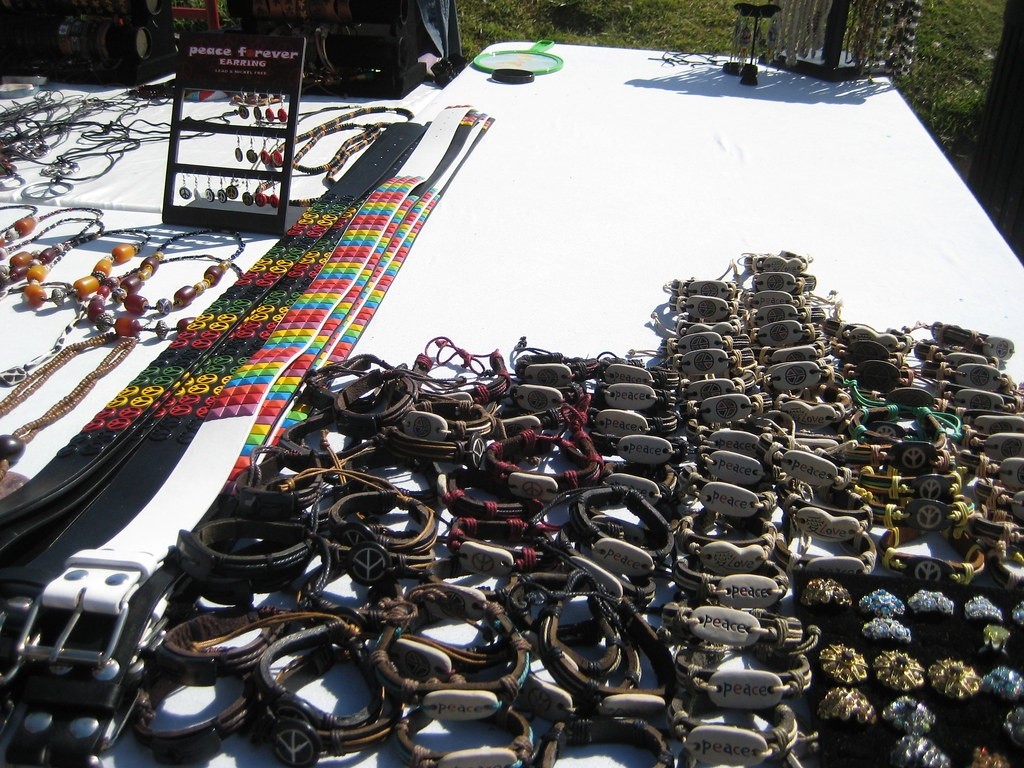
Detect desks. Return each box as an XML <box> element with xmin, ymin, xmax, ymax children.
<box><xmin>0</xmin><ymin>39</ymin><xmax>1023</xmax><ymax>768</ymax></box>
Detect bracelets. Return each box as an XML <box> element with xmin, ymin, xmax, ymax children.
<box><xmin>1</xmin><ymin>0</ymin><xmax>360</xmax><ymax>89</ymax></box>
<box><xmin>123</xmin><ymin>237</ymin><xmax>1024</xmax><ymax>768</ymax></box>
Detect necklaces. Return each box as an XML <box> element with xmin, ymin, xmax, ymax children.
<box><xmin>0</xmin><ymin>60</ymin><xmax>423</xmax><ymax>497</ymax></box>
<box><xmin>764</xmin><ymin>0</ymin><xmax>923</xmax><ymax>88</ymax></box>
